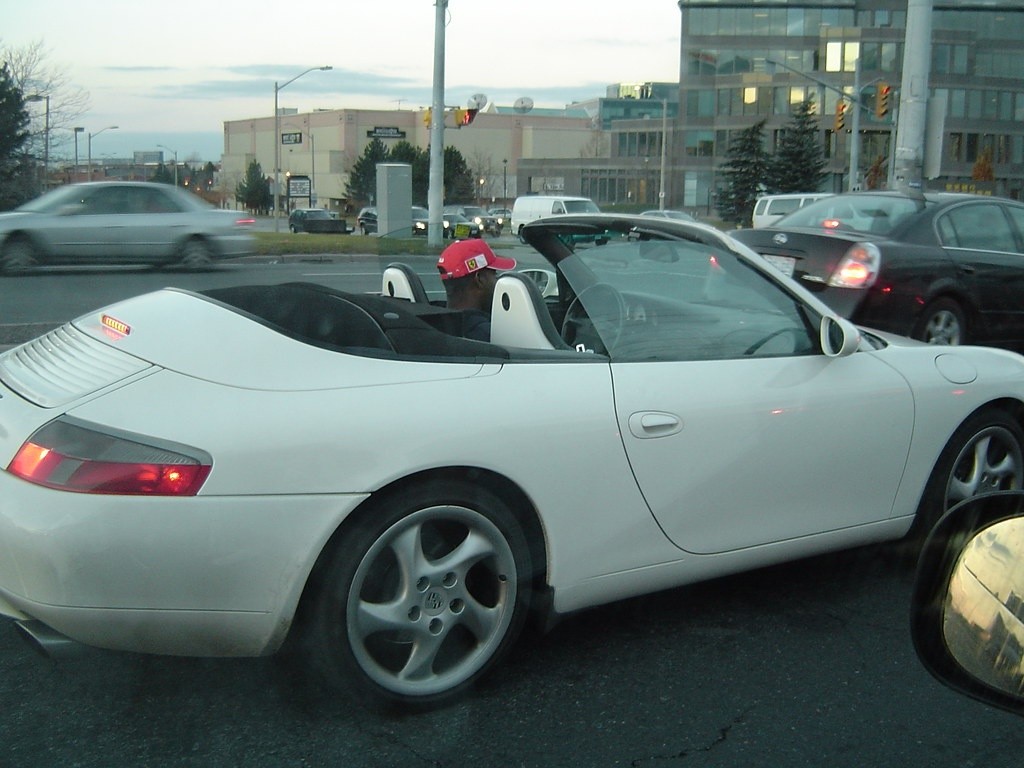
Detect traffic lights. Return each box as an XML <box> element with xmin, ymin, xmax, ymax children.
<box><xmin>835</xmin><ymin>100</ymin><xmax>847</xmax><ymax>130</ymax></box>
<box><xmin>877</xmin><ymin>83</ymin><xmax>891</xmax><ymax>119</ymax></box>
<box><xmin>457</xmin><ymin>110</ymin><xmax>478</xmax><ymax>125</ymax></box>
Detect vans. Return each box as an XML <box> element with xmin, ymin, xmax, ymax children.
<box><xmin>510</xmin><ymin>195</ymin><xmax>612</xmax><ymax>246</ymax></box>
<box><xmin>751</xmin><ymin>195</ymin><xmax>874</xmax><ymax>233</ymax></box>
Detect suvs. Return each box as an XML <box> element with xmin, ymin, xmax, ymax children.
<box><xmin>443</xmin><ymin>206</ymin><xmax>504</xmax><ymax>237</ymax></box>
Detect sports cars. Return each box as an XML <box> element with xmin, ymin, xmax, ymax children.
<box><xmin>0</xmin><ymin>211</ymin><xmax>1023</xmax><ymax>713</ymax></box>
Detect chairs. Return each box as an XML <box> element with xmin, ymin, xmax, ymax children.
<box><xmin>380</xmin><ymin>262</ymin><xmax>429</xmax><ymax>304</ymax></box>
<box><xmin>490</xmin><ymin>271</ymin><xmax>576</xmax><ymax>351</ymax></box>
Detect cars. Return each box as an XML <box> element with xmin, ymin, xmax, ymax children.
<box><xmin>358</xmin><ymin>207</ymin><xmax>378</xmax><ymax>235</ymax></box>
<box><xmin>412</xmin><ymin>206</ymin><xmax>450</xmax><ymax>239</ymax></box>
<box><xmin>1</xmin><ymin>181</ymin><xmax>255</xmax><ymax>276</ymax></box>
<box><xmin>487</xmin><ymin>208</ymin><xmax>512</xmax><ymax>222</ymax></box>
<box><xmin>288</xmin><ymin>208</ymin><xmax>355</xmax><ymax>235</ymax></box>
<box><xmin>629</xmin><ymin>211</ymin><xmax>716</xmax><ymax>240</ymax></box>
<box><xmin>722</xmin><ymin>193</ymin><xmax>1023</xmax><ymax>344</ymax></box>
<box><xmin>443</xmin><ymin>213</ymin><xmax>484</xmax><ymax>240</ymax></box>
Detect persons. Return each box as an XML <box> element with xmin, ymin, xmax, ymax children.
<box><xmin>436</xmin><ymin>238</ymin><xmax>517</xmax><ymax>343</ymax></box>
<box><xmin>863</xmin><ymin>160</ymin><xmax>883</xmax><ymax>190</ymax></box>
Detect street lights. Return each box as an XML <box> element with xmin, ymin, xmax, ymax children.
<box><xmin>766</xmin><ymin>57</ymin><xmax>897</xmax><ymax>194</ymax></box>
<box><xmin>634</xmin><ymin>85</ymin><xmax>668</xmax><ymax>210</ymax></box>
<box><xmin>848</xmin><ymin>59</ymin><xmax>885</xmax><ymax>194</ymax></box>
<box><xmin>156</xmin><ymin>144</ymin><xmax>178</xmax><ymax>186</ymax></box>
<box><xmin>273</xmin><ymin>64</ymin><xmax>334</xmax><ymax>232</ymax></box>
<box><xmin>88</xmin><ymin>125</ymin><xmax>120</xmax><ymax>183</ymax></box>
<box><xmin>283</xmin><ymin>122</ymin><xmax>315</xmax><ymax>207</ymax></box>
<box><xmin>26</xmin><ymin>95</ymin><xmax>50</xmax><ymax>195</ymax></box>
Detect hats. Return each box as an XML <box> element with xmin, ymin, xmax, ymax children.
<box><xmin>436</xmin><ymin>238</ymin><xmax>517</xmax><ymax>281</ymax></box>
